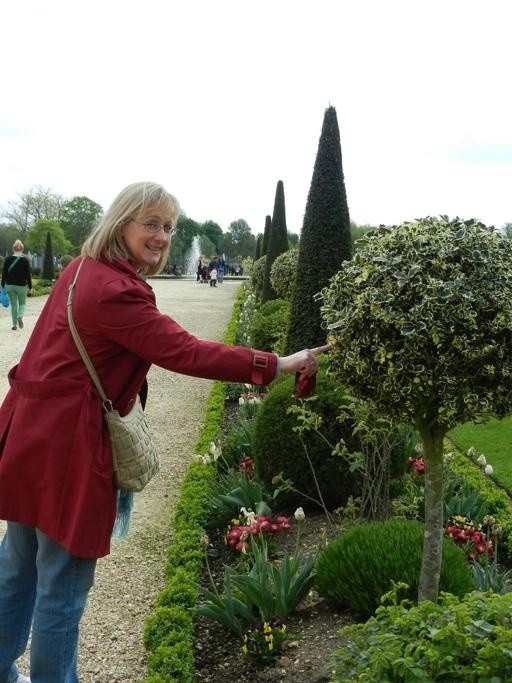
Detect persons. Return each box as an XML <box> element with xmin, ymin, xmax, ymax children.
<box><xmin>195</xmin><ymin>253</ymin><xmax>244</xmax><ymax>287</ymax></box>
<box><xmin>1</xmin><ymin>239</ymin><xmax>34</xmax><ymax>330</ymax></box>
<box><xmin>0</xmin><ymin>181</ymin><xmax>334</xmax><ymax>683</ymax></box>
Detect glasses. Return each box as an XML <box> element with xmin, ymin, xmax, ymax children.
<box><xmin>130</xmin><ymin>216</ymin><xmax>175</xmax><ymax>234</ymax></box>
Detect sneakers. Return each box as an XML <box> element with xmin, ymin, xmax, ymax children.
<box><xmin>17</xmin><ymin>317</ymin><xmax>23</xmax><ymax>328</ymax></box>
<box><xmin>11</xmin><ymin>327</ymin><xmax>16</xmax><ymax>330</ymax></box>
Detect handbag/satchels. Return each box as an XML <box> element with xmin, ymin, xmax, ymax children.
<box><xmin>100</xmin><ymin>394</ymin><xmax>160</xmax><ymax>492</ymax></box>
<box><xmin>0</xmin><ymin>288</ymin><xmax>9</xmax><ymax>307</ymax></box>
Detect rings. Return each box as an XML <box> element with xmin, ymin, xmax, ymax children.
<box><xmin>311</xmin><ymin>360</ymin><xmax>315</xmax><ymax>366</ymax></box>
